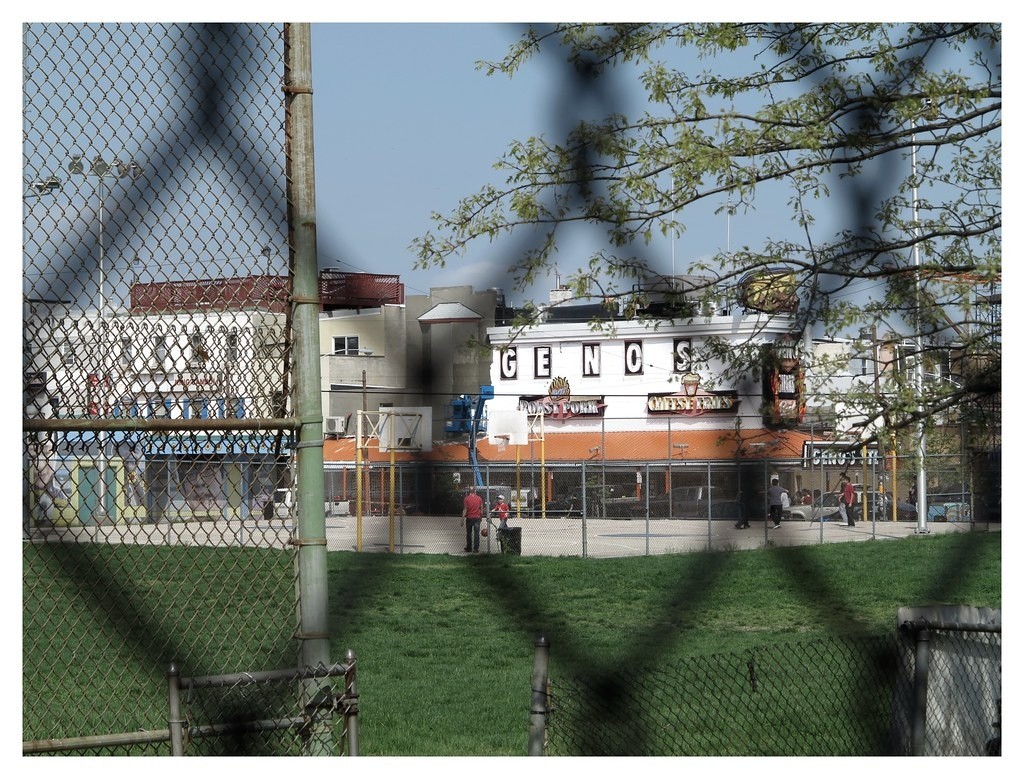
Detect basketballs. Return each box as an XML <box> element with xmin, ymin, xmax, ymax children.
<box><xmin>481</xmin><ymin>529</ymin><xmax>488</xmax><ymax>537</ymax></box>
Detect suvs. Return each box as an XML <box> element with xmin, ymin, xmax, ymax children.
<box><xmin>779</xmin><ymin>483</ymin><xmax>893</xmax><ymax>521</ymax></box>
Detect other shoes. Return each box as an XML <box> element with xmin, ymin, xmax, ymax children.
<box><xmin>774</xmin><ymin>525</ymin><xmax>780</xmax><ymax>529</ymax></box>
<box><xmin>839</xmin><ymin>523</ymin><xmax>848</xmax><ymax>525</ymax></box>
<box><xmin>473</xmin><ymin>550</ymin><xmax>479</xmax><ymax>553</ymax></box>
<box><xmin>464</xmin><ymin>548</ymin><xmax>471</xmax><ymax>552</ymax></box>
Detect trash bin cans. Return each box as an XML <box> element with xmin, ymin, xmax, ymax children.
<box><xmin>497</xmin><ymin>527</ymin><xmax>522</xmax><ymax>555</ymax></box>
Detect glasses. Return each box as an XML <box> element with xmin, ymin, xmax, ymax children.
<box><xmin>181</xmin><ymin>465</ymin><xmax>221</xmax><ymax>483</ymax></box>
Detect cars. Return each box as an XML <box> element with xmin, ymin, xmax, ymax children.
<box><xmin>814</xmin><ymin>500</ymin><xmax>918</xmax><ymax>522</ymax></box>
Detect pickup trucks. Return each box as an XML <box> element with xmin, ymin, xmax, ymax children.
<box><xmin>653</xmin><ymin>485</ymin><xmax>738</xmax><ymax>517</ymax></box>
<box><xmin>534</xmin><ymin>484</ymin><xmax>674</xmax><ymax>519</ymax></box>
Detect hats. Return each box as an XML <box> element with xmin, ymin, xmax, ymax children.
<box><xmin>496</xmin><ymin>495</ymin><xmax>504</xmax><ymax>499</ymax></box>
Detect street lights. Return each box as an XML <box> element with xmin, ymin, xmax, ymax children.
<box><xmin>910</xmin><ymin>99</ymin><xmax>939</xmax><ymax>536</ymax></box>
<box><xmin>320</xmin><ymin>349</ymin><xmax>374</xmax><ymax>360</ymax></box>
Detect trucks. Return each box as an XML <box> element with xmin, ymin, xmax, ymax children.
<box><xmin>273</xmin><ymin>488</ymin><xmax>351</xmax><ymax>519</ymax></box>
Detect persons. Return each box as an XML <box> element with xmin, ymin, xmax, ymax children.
<box><xmin>838</xmin><ymin>472</ymin><xmax>850</xmax><ymax>526</ymax></box>
<box><xmin>177</xmin><ymin>452</ymin><xmax>225</xmax><ymax>522</ymax></box>
<box><xmin>767</xmin><ymin>479</ymin><xmax>789</xmax><ymax>529</ymax></box>
<box><xmin>460</xmin><ymin>486</ymin><xmax>483</xmax><ymax>553</ymax></box>
<box><xmin>734</xmin><ymin>485</ymin><xmax>751</xmax><ymax>529</ymax></box>
<box><xmin>839</xmin><ymin>476</ymin><xmax>856</xmax><ymax>527</ymax></box>
<box><xmin>25</xmin><ymin>430</ymin><xmax>65</xmax><ymax>521</ymax></box>
<box><xmin>911</xmin><ymin>475</ymin><xmax>918</xmax><ymax>514</ymax></box>
<box><xmin>109</xmin><ymin>440</ymin><xmax>146</xmax><ymax>508</ymax></box>
<box><xmin>489</xmin><ymin>495</ymin><xmax>510</xmax><ymax>541</ymax></box>
<box><xmin>788</xmin><ymin>488</ymin><xmax>821</xmax><ymax>521</ymax></box>
